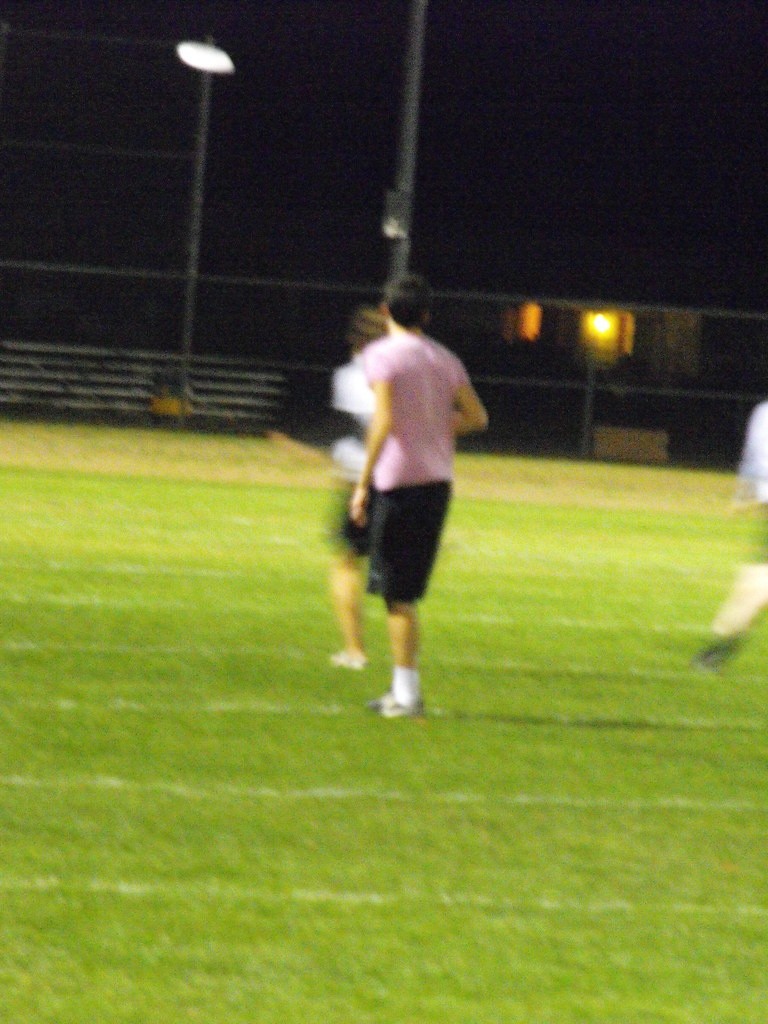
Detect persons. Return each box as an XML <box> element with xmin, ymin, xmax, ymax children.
<box><xmin>700</xmin><ymin>404</ymin><xmax>768</xmax><ymax>674</ymax></box>
<box><xmin>325</xmin><ymin>278</ymin><xmax>489</xmax><ymax>722</ymax></box>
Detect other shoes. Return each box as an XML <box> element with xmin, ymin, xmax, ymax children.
<box><xmin>365</xmin><ymin>690</ymin><xmax>425</xmax><ymax>720</ymax></box>
<box><xmin>330</xmin><ymin>646</ymin><xmax>372</xmax><ymax>672</ymax></box>
